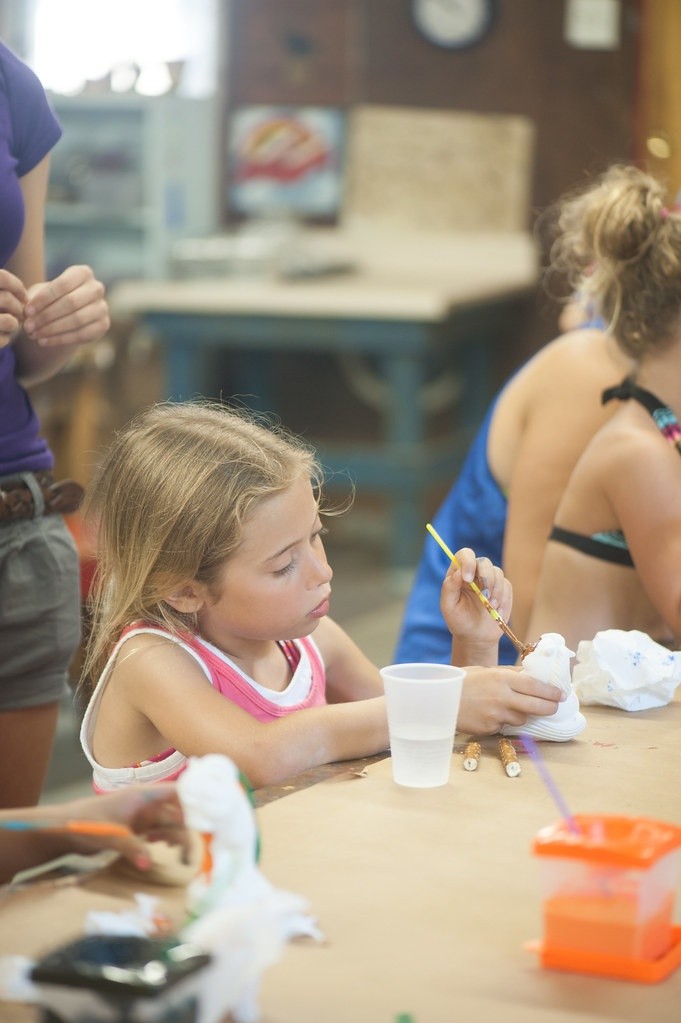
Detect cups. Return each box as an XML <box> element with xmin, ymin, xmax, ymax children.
<box><xmin>380</xmin><ymin>662</ymin><xmax>466</xmax><ymax>788</ymax></box>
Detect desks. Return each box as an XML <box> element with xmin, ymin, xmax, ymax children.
<box><xmin>110</xmin><ymin>231</ymin><xmax>543</xmax><ymax>578</ymax></box>
<box><xmin>0</xmin><ymin>707</ymin><xmax>681</xmax><ymax>1023</ymax></box>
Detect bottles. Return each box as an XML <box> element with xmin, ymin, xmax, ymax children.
<box><xmin>529</xmin><ymin>810</ymin><xmax>681</xmax><ymax>984</ymax></box>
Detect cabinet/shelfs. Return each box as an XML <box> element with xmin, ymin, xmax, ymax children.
<box><xmin>45</xmin><ymin>96</ymin><xmax>218</xmax><ymax>291</ymax></box>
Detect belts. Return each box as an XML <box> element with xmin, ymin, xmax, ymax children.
<box><xmin>0</xmin><ymin>474</ymin><xmax>85</xmax><ymax>527</ymax></box>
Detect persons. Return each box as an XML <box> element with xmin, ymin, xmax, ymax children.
<box><xmin>0</xmin><ymin>43</ymin><xmax>109</xmax><ymax>811</ymax></box>
<box><xmin>72</xmin><ymin>402</ymin><xmax>567</xmax><ymax>825</ymax></box>
<box><xmin>395</xmin><ymin>271</ymin><xmax>637</xmax><ymax>666</ymax></box>
<box><xmin>0</xmin><ymin>785</ymin><xmax>188</xmax><ymax>885</ymax></box>
<box><xmin>521</xmin><ymin>167</ymin><xmax>681</xmax><ymax>649</ymax></box>
